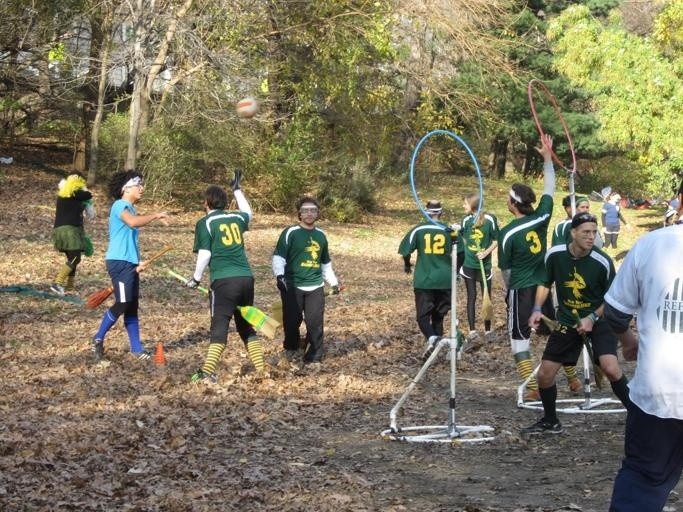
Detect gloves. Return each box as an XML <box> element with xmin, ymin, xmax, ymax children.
<box><xmin>278</xmin><ymin>276</ymin><xmax>287</xmax><ymax>293</ymax></box>
<box><xmin>230</xmin><ymin>169</ymin><xmax>241</xmax><ymax>190</ymax></box>
<box><xmin>404</xmin><ymin>264</ymin><xmax>412</xmax><ymax>274</ymax></box>
<box><xmin>185</xmin><ymin>279</ymin><xmax>200</xmax><ymax>289</ymax></box>
<box><xmin>332</xmin><ymin>285</ymin><xmax>339</xmax><ymax>293</ymax></box>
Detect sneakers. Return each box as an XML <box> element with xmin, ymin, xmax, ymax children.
<box><xmin>91</xmin><ymin>339</ymin><xmax>103</xmax><ymax>358</ymax></box>
<box><xmin>521</xmin><ymin>418</ymin><xmax>563</xmax><ymax>434</ymax></box>
<box><xmin>128</xmin><ymin>352</ymin><xmax>151</xmax><ymax>361</ymax></box>
<box><xmin>49</xmin><ymin>284</ymin><xmax>64</xmax><ymax>296</ymax></box>
<box><xmin>421</xmin><ymin>335</ymin><xmax>440</xmax><ymax>360</ymax></box>
<box><xmin>568</xmin><ymin>380</ymin><xmax>582</xmax><ymax>392</ymax></box>
<box><xmin>523</xmin><ymin>391</ymin><xmax>539</xmax><ymax>401</ymax></box>
<box><xmin>192</xmin><ymin>371</ymin><xmax>217</xmax><ymax>385</ymax></box>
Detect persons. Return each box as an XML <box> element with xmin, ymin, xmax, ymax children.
<box><xmin>601</xmin><ymin>189</ymin><xmax>633</xmax><ymax>254</ymax></box>
<box><xmin>186</xmin><ymin>170</ymin><xmax>265</xmax><ymax>381</ymax></box>
<box><xmin>663</xmin><ymin>196</ymin><xmax>679</xmax><ymax>225</ymax></box>
<box><xmin>519</xmin><ymin>213</ymin><xmax>632</xmax><ymax>435</ymax></box>
<box><xmin>551</xmin><ymin>194</ymin><xmax>577</xmax><ymax>246</ymax></box>
<box><xmin>50</xmin><ymin>172</ymin><xmax>97</xmax><ymax>299</ymax></box>
<box><xmin>460</xmin><ymin>196</ymin><xmax>499</xmax><ymax>337</ymax></box>
<box><xmin>604</xmin><ymin>183</ymin><xmax>683</xmax><ymax>511</ymax></box>
<box><xmin>398</xmin><ymin>200</ymin><xmax>466</xmax><ymax>363</ymax></box>
<box><xmin>90</xmin><ymin>170</ymin><xmax>175</xmax><ymax>361</ymax></box>
<box><xmin>271</xmin><ymin>196</ymin><xmax>340</xmax><ymax>361</ymax></box>
<box><xmin>499</xmin><ymin>133</ymin><xmax>583</xmax><ymax>401</ymax></box>
<box><xmin>561</xmin><ymin>195</ymin><xmax>604</xmax><ymax>247</ymax></box>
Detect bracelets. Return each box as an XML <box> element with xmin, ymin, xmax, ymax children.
<box><xmin>532</xmin><ymin>305</ymin><xmax>542</xmax><ymax>311</ymax></box>
<box><xmin>589</xmin><ymin>312</ymin><xmax>599</xmax><ymax>321</ymax></box>
